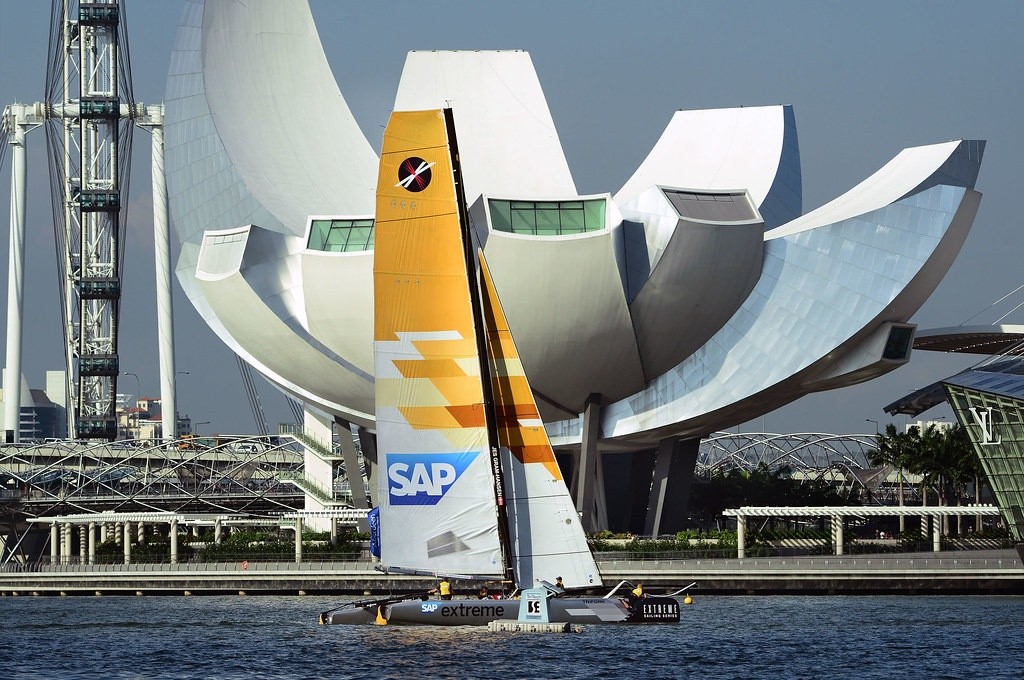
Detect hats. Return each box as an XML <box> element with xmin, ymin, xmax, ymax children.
<box><xmin>556</xmin><ymin>577</ymin><xmax>562</xmax><ymax>580</ymax></box>
<box><xmin>444</xmin><ymin>577</ymin><xmax>449</xmax><ymax>582</ymax></box>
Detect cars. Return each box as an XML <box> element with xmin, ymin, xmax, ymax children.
<box><xmin>232</xmin><ymin>444</ymin><xmax>258</xmax><ymax>454</ymax></box>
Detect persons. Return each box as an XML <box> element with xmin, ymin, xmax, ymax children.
<box><xmin>478</xmin><ymin>587</ymin><xmax>488</xmax><ymax>599</ymax></box>
<box><xmin>556</xmin><ymin>577</ymin><xmax>565</xmax><ymax>598</ymax></box>
<box><xmin>428</xmin><ymin>577</ymin><xmax>456</xmax><ymax>600</ymax></box>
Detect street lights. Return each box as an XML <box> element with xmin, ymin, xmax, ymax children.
<box><xmin>866</xmin><ymin>419</ymin><xmax>879</xmax><ymax>470</ymax></box>
<box><xmin>172</xmin><ymin>371</ymin><xmax>191</xmax><ymax>452</ymax></box>
<box><xmin>193</xmin><ymin>420</ymin><xmax>210</xmax><ymax>438</ymax></box>
<box><xmin>904</xmin><ymin>388</ymin><xmax>918</xmax><ymax>435</ymax></box>
<box><xmin>931</xmin><ymin>416</ymin><xmax>946</xmax><ymax>436</ymax></box>
<box><xmin>124</xmin><ymin>372</ymin><xmax>140</xmax><ymax>443</ymax></box>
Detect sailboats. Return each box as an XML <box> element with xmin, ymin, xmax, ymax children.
<box><xmin>316</xmin><ymin>105</ymin><xmax>699</xmax><ymax>638</ymax></box>
